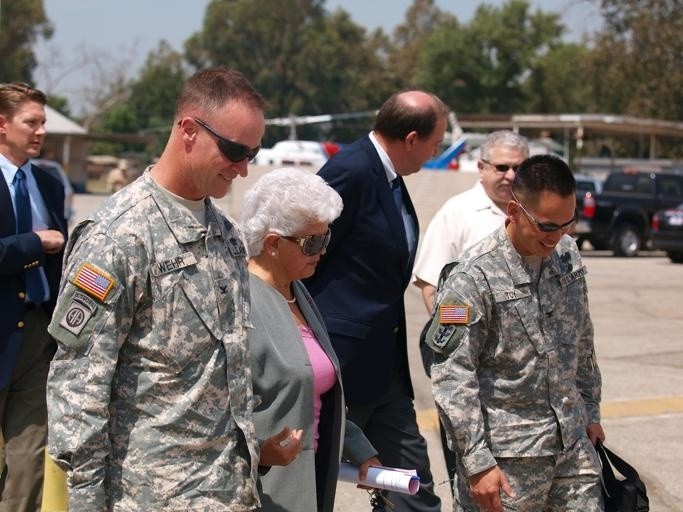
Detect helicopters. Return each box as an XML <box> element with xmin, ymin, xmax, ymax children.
<box><xmin>140</xmin><ymin>111</ymin><xmax>469</xmax><ymax>174</ymax></box>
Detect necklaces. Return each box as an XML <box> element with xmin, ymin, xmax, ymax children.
<box><xmin>286</xmin><ymin>297</ymin><xmax>297</xmax><ymax>304</ymax></box>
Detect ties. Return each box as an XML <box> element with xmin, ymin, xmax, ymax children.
<box><xmin>393</xmin><ymin>174</ymin><xmax>405</xmax><ymax>216</ymax></box>
<box><xmin>12</xmin><ymin>169</ymin><xmax>46</xmax><ymax>307</ymax></box>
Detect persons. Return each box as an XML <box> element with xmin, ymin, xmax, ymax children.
<box><xmin>420</xmin><ymin>154</ymin><xmax>606</xmax><ymax>511</ymax></box>
<box><xmin>0</xmin><ymin>80</ymin><xmax>68</xmax><ymax>512</ymax></box>
<box><xmin>298</xmin><ymin>88</ymin><xmax>447</xmax><ymax>512</ymax></box>
<box><xmin>46</xmin><ymin>66</ymin><xmax>265</xmax><ymax>512</ymax></box>
<box><xmin>412</xmin><ymin>128</ymin><xmax>532</xmax><ymax>496</ymax></box>
<box><xmin>241</xmin><ymin>168</ymin><xmax>385</xmax><ymax>512</ymax></box>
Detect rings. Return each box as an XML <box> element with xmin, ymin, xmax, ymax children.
<box><xmin>294</xmin><ymin>453</ymin><xmax>300</xmax><ymax>460</ymax></box>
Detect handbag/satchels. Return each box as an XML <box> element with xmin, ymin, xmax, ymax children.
<box><xmin>594</xmin><ymin>438</ymin><xmax>648</xmax><ymax>511</ymax></box>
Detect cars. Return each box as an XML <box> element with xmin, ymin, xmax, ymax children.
<box><xmin>648</xmin><ymin>196</ymin><xmax>682</xmax><ymax>262</ymax></box>
<box><xmin>572</xmin><ymin>177</ymin><xmax>599</xmax><ymax>249</ymax></box>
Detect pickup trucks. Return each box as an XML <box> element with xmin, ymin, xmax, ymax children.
<box><xmin>573</xmin><ymin>171</ymin><xmax>677</xmax><ymax>256</ymax></box>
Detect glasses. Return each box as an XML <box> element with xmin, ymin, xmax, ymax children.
<box><xmin>280</xmin><ymin>228</ymin><xmax>332</xmax><ymax>256</ymax></box>
<box><xmin>519</xmin><ymin>204</ymin><xmax>579</xmax><ymax>234</ymax></box>
<box><xmin>177</xmin><ymin>116</ymin><xmax>261</xmax><ymax>163</ymax></box>
<box><xmin>483</xmin><ymin>159</ymin><xmax>518</xmax><ymax>173</ymax></box>
